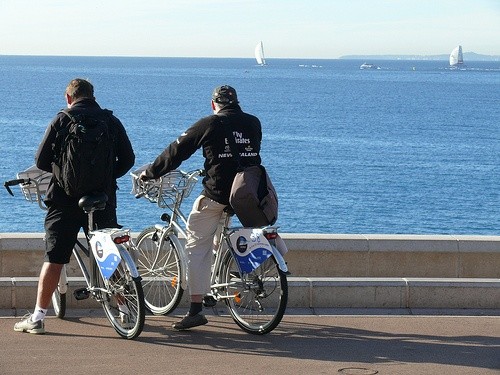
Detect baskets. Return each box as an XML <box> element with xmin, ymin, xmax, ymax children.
<box><xmin>129</xmin><ymin>162</ymin><xmax>199</xmax><ymax>203</ymax></box>
<box><xmin>16</xmin><ymin>164</ymin><xmax>53</xmax><ymax>202</ymax></box>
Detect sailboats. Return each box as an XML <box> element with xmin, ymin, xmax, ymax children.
<box><xmin>254</xmin><ymin>41</ymin><xmax>269</xmax><ymax>67</ymax></box>
<box><xmin>450</xmin><ymin>46</ymin><xmax>464</xmax><ymax>66</ymax></box>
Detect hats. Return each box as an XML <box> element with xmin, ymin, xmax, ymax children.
<box><xmin>212</xmin><ymin>85</ymin><xmax>239</xmax><ymax>104</ymax></box>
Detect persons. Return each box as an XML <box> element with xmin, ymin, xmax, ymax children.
<box><xmin>14</xmin><ymin>79</ymin><xmax>136</xmax><ymax>335</ymax></box>
<box><xmin>139</xmin><ymin>84</ymin><xmax>263</xmax><ymax>329</ymax></box>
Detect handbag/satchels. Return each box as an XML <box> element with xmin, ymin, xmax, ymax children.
<box><xmin>229</xmin><ymin>164</ymin><xmax>278</xmax><ymax>228</ymax></box>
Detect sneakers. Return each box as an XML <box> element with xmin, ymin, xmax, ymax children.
<box><xmin>119</xmin><ymin>310</ymin><xmax>135</xmax><ymax>329</ymax></box>
<box><xmin>171</xmin><ymin>310</ymin><xmax>208</xmax><ymax>330</ymax></box>
<box><xmin>14</xmin><ymin>309</ymin><xmax>46</xmax><ymax>334</ymax></box>
<box><xmin>203</xmin><ymin>296</ymin><xmax>217</xmax><ymax>307</ymax></box>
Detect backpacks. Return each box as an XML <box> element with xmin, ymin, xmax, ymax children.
<box><xmin>57</xmin><ymin>107</ymin><xmax>120</xmax><ymax>199</ymax></box>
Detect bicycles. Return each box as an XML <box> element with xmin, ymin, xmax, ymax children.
<box><xmin>3</xmin><ymin>162</ymin><xmax>148</xmax><ymax>338</ymax></box>
<box><xmin>130</xmin><ymin>161</ymin><xmax>293</xmax><ymax>336</ymax></box>
